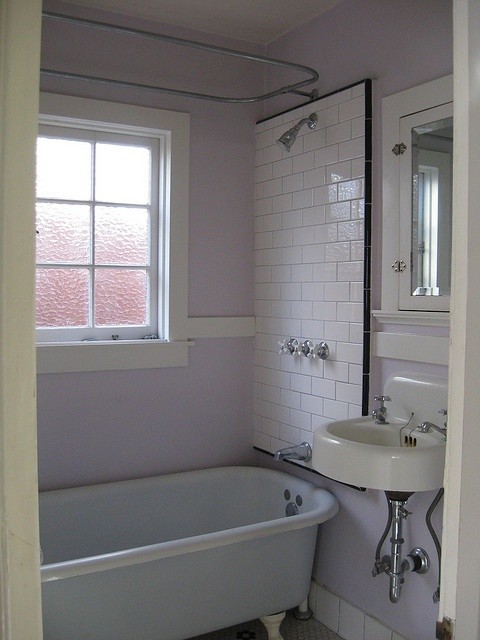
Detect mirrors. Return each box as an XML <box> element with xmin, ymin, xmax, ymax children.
<box><xmin>398</xmin><ymin>99</ymin><xmax>450</xmax><ymax>314</ymax></box>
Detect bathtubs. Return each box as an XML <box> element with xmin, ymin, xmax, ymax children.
<box><xmin>38</xmin><ymin>468</ymin><xmax>340</xmax><ymax>638</ymax></box>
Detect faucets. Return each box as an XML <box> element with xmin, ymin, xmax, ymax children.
<box><xmin>275</xmin><ymin>442</ymin><xmax>310</xmax><ymax>464</ymax></box>
<box><xmin>370</xmin><ymin>395</ymin><xmax>391</xmax><ymax>423</ymax></box>
<box><xmin>421</xmin><ymin>409</ymin><xmax>446</xmax><ymax>439</ymax></box>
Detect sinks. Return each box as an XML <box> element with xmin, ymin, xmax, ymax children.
<box><xmin>309</xmin><ymin>421</ymin><xmax>446</xmax><ymax>490</ymax></box>
